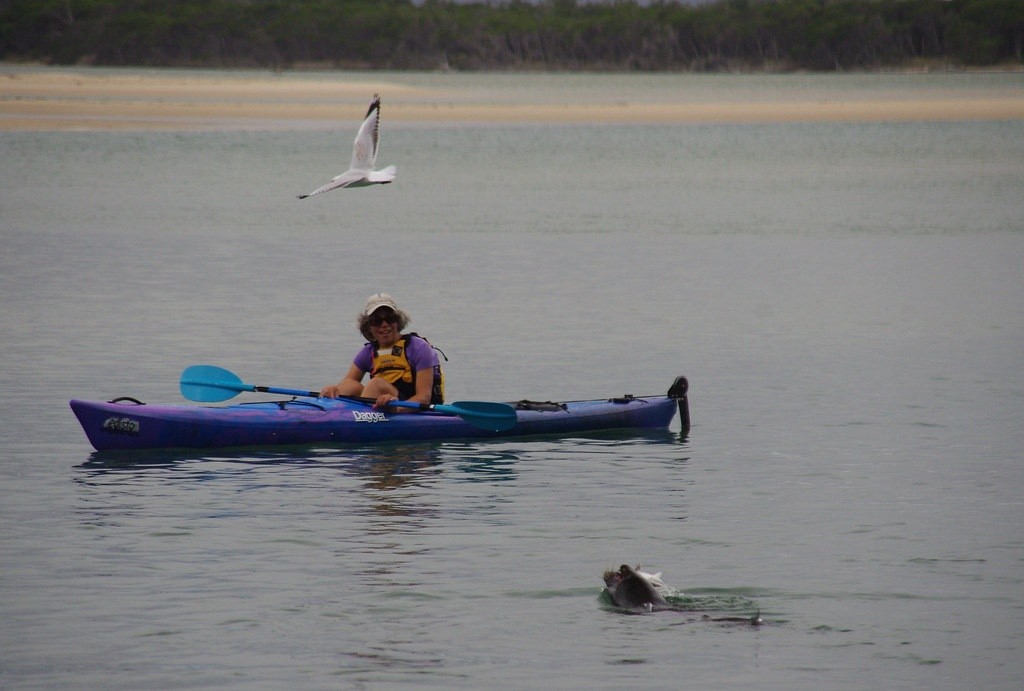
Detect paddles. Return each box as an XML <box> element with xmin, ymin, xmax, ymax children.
<box><xmin>179</xmin><ymin>364</ymin><xmax>518</xmax><ymax>433</ymax></box>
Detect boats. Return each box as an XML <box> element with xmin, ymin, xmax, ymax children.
<box><xmin>70</xmin><ymin>376</ymin><xmax>692</xmax><ymax>449</ymax></box>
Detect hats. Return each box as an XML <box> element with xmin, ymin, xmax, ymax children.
<box><xmin>364</xmin><ymin>294</ymin><xmax>398</xmax><ymax>315</ymax></box>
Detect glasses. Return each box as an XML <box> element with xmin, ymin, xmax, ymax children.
<box><xmin>367</xmin><ymin>313</ymin><xmax>398</xmax><ymax>327</ymax></box>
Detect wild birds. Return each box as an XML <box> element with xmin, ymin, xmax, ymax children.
<box><xmin>295</xmin><ymin>91</ymin><xmax>398</xmax><ymax>199</ymax></box>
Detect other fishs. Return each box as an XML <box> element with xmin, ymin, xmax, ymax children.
<box><xmin>604</xmin><ymin>564</ymin><xmax>671</xmax><ymax>614</ymax></box>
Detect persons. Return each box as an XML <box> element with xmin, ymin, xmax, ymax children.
<box><xmin>319</xmin><ymin>294</ymin><xmax>445</xmax><ymax>414</ymax></box>
